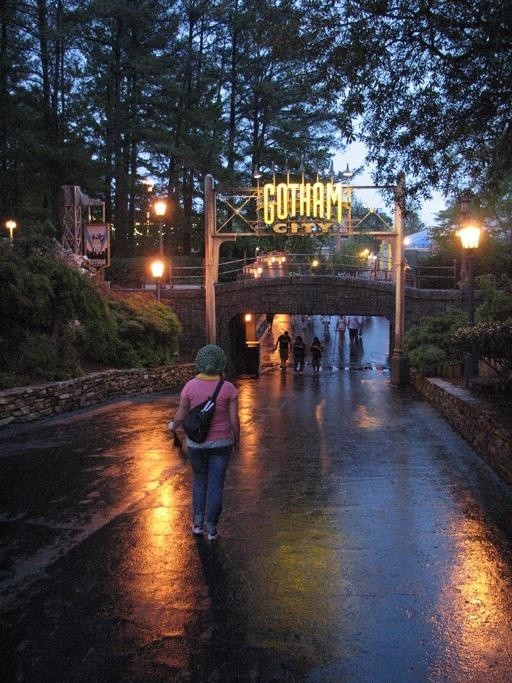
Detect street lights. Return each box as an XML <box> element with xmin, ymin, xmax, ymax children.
<box><xmin>456</xmin><ymin>188</ymin><xmax>481</xmax><ymax>378</ymax></box>
<box><xmin>5</xmin><ymin>219</ymin><xmax>17</xmax><ymax>244</ymax></box>
<box><xmin>140</xmin><ymin>178</ymin><xmax>166</xmax><ymax>304</ymax></box>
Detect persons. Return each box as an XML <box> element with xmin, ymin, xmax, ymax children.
<box><xmin>274</xmin><ymin>331</ymin><xmax>292</xmax><ymax>371</ymax></box>
<box><xmin>293</xmin><ymin>336</ymin><xmax>306</xmax><ymax>377</ymax></box>
<box><xmin>319</xmin><ymin>313</ymin><xmax>364</xmax><ymax>345</ymax></box>
<box><xmin>310</xmin><ymin>336</ymin><xmax>323</xmax><ymax>370</ymax></box>
<box><xmin>167</xmin><ymin>343</ymin><xmax>240</xmax><ymax>540</ymax></box>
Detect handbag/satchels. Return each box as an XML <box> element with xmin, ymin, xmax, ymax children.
<box><xmin>182</xmin><ymin>396</ymin><xmax>216</xmax><ymax>443</ymax></box>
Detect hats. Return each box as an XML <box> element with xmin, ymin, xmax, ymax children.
<box><xmin>195</xmin><ymin>344</ymin><xmax>226</xmax><ymax>376</ymax></box>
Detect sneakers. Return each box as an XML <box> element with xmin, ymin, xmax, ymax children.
<box><xmin>192</xmin><ymin>523</ymin><xmax>204</xmax><ymax>535</ymax></box>
<box><xmin>208</xmin><ymin>529</ymin><xmax>219</xmax><ymax>540</ymax></box>
